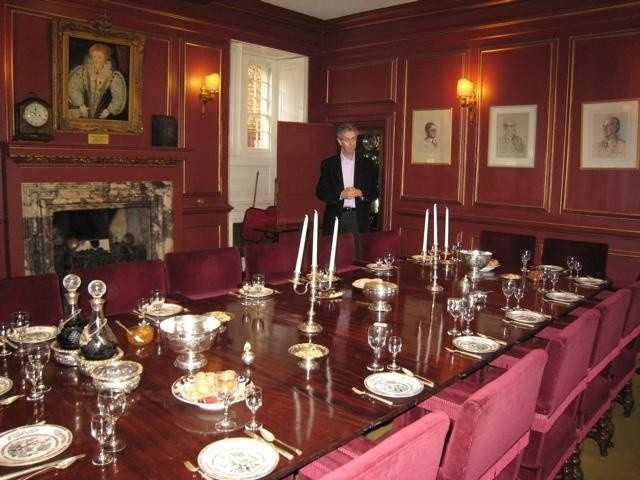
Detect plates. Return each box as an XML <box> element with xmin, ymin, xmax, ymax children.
<box><xmin>307</xmin><ymin>274</ymin><xmax>339</xmax><ymax>282</ymax></box>
<box><xmin>538</xmin><ymin>264</ymin><xmax>565</xmax><ymax>272</ymax></box>
<box><xmin>199</xmin><ymin>436</ymin><xmax>280</xmax><ymax>480</ymax></box>
<box><xmin>366</xmin><ymin>264</ymin><xmax>393</xmax><ymax>271</ymax></box>
<box><xmin>576</xmin><ymin>277</ymin><xmax>605</xmax><ymax>286</ymax></box>
<box><xmin>239</xmin><ymin>286</ymin><xmax>273</xmax><ymax>297</ymax></box>
<box><xmin>505</xmin><ymin>310</ymin><xmax>546</xmax><ymax>324</ymax></box>
<box><xmin>364</xmin><ymin>373</ymin><xmax>426</xmax><ymax>401</ymax></box>
<box><xmin>452</xmin><ymin>336</ymin><xmax>501</xmax><ymax>353</ymax></box>
<box><xmin>411</xmin><ymin>255</ymin><xmax>441</xmax><ymax>261</ymax></box>
<box><xmin>545</xmin><ymin>292</ymin><xmax>580</xmax><ymax>303</ymax></box>
<box><xmin>172</xmin><ymin>373</ymin><xmax>255</xmax><ymax>410</ymax></box>
<box><xmin>0</xmin><ymin>425</ymin><xmax>82</xmax><ymax>466</ymax></box>
<box><xmin>138</xmin><ymin>303</ymin><xmax>184</xmax><ymax>318</ymax></box>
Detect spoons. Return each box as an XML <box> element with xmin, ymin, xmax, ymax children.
<box><xmin>259</xmin><ymin>427</ymin><xmax>303</xmax><ymax>456</ymax></box>
<box><xmin>19</xmin><ymin>457</ymin><xmax>78</xmax><ymax>480</ymax></box>
<box><xmin>402</xmin><ymin>366</ymin><xmax>434</xmax><ymax>385</ymax></box>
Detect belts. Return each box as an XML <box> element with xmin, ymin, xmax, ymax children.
<box><xmin>342</xmin><ymin>206</ymin><xmax>356</xmax><ymax>214</ymax></box>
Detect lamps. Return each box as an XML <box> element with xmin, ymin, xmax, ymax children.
<box><xmin>457</xmin><ymin>78</ymin><xmax>477</xmax><ymax>126</ymax></box>
<box><xmin>199</xmin><ymin>73</ymin><xmax>221</xmax><ymax>119</ymax></box>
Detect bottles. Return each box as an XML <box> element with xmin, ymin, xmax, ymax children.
<box><xmin>56</xmin><ymin>274</ymin><xmax>87</xmax><ymax>349</ymax></box>
<box><xmin>80</xmin><ymin>280</ymin><xmax>117</xmax><ymax>360</ymax></box>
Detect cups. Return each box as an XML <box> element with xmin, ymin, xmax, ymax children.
<box><xmin>325</xmin><ymin>263</ymin><xmax>337</xmax><ymax>276</ymax></box>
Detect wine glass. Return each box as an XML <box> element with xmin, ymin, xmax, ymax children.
<box><xmin>513</xmin><ymin>288</ymin><xmax>524</xmax><ymax>310</ymax></box>
<box><xmin>539</xmin><ymin>266</ymin><xmax>551</xmax><ymax>293</ymax></box>
<box><xmin>215</xmin><ymin>372</ymin><xmax>239</xmax><ymax>433</ymax></box>
<box><xmin>550</xmin><ymin>274</ymin><xmax>560</xmax><ymax>292</ymax></box>
<box><xmin>150</xmin><ymin>289</ymin><xmax>166</xmax><ymax>328</ymax></box>
<box><xmin>251</xmin><ymin>273</ymin><xmax>266</xmax><ymax>309</ymax></box>
<box><xmin>98</xmin><ymin>388</ymin><xmax>127</xmax><ymax>452</ymax></box>
<box><xmin>366</xmin><ymin>325</ymin><xmax>387</xmax><ymax>371</ymax></box>
<box><xmin>384</xmin><ymin>253</ymin><xmax>395</xmax><ymax>276</ymax></box>
<box><xmin>387</xmin><ymin>335</ymin><xmax>403</xmax><ymax>371</ymax></box>
<box><xmin>375</xmin><ymin>256</ymin><xmax>383</xmax><ymax>277</ymax></box>
<box><xmin>501</xmin><ymin>280</ymin><xmax>515</xmax><ymax>311</ymax></box>
<box><xmin>0</xmin><ymin>312</ymin><xmax>58</xmax><ymax>401</ymax></box>
<box><xmin>90</xmin><ymin>414</ymin><xmax>114</xmax><ymax>467</ymax></box>
<box><xmin>566</xmin><ymin>256</ymin><xmax>576</xmax><ymax>279</ymax></box>
<box><xmin>574</xmin><ymin>261</ymin><xmax>582</xmax><ymax>277</ymax></box>
<box><xmin>521</xmin><ymin>249</ymin><xmax>531</xmax><ymax>272</ymax></box>
<box><xmin>463</xmin><ymin>307</ymin><xmax>475</xmax><ymax>335</ymax></box>
<box><xmin>137</xmin><ymin>298</ymin><xmax>151</xmax><ymax>327</ymax></box>
<box><xmin>447</xmin><ymin>298</ymin><xmax>464</xmax><ymax>336</ymax></box>
<box><xmin>241</xmin><ymin>281</ymin><xmax>251</xmax><ymax>306</ymax></box>
<box><xmin>243</xmin><ymin>385</ymin><xmax>263</xmax><ymax>431</ymax></box>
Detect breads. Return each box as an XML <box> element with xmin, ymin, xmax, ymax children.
<box><xmin>188</xmin><ymin>370</ymin><xmax>237</xmax><ymax>396</ymax></box>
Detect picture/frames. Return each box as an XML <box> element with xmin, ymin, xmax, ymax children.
<box><xmin>51</xmin><ymin>16</ymin><xmax>145</xmax><ymax>135</ymax></box>
<box><xmin>487</xmin><ymin>105</ymin><xmax>538</xmax><ymax>168</ymax></box>
<box><xmin>411</xmin><ymin>107</ymin><xmax>452</xmax><ymax>166</ymax></box>
<box><xmin>580</xmin><ymin>99</ymin><xmax>640</xmax><ymax>171</ymax></box>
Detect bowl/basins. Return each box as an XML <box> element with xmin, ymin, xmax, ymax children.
<box><xmin>50</xmin><ymin>342</ymin><xmax>143</xmax><ymax>393</ymax></box>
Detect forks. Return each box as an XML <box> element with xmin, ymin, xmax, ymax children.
<box><xmin>350</xmin><ymin>387</ymin><xmax>393</xmax><ymax>406</ymax></box>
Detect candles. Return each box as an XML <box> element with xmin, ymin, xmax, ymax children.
<box><xmin>294</xmin><ymin>213</ymin><xmax>309</xmax><ymax>274</ymax></box>
<box><xmin>445</xmin><ymin>207</ymin><xmax>449</xmax><ymax>247</ymax></box>
<box><xmin>433</xmin><ymin>205</ymin><xmax>438</xmax><ymax>243</ymax></box>
<box><xmin>329</xmin><ymin>216</ymin><xmax>339</xmax><ymax>271</ymax></box>
<box><xmin>422</xmin><ymin>209</ymin><xmax>429</xmax><ymax>250</ymax></box>
<box><xmin>312</xmin><ymin>210</ymin><xmax>319</xmax><ymax>266</ymax></box>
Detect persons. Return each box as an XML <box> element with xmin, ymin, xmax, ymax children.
<box><xmin>593</xmin><ymin>116</ymin><xmax>626</xmax><ymax>158</ymax></box>
<box><xmin>422</xmin><ymin>123</ymin><xmax>440</xmax><ymax>149</ymax></box>
<box><xmin>68</xmin><ymin>41</ymin><xmax>129</xmax><ymax>120</ymax></box>
<box><xmin>314</xmin><ymin>122</ymin><xmax>380</xmax><ymax>236</ymax></box>
<box><xmin>496</xmin><ymin>118</ymin><xmax>526</xmax><ymax>158</ymax></box>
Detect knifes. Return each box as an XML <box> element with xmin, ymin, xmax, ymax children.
<box><xmin>242</xmin><ymin>430</ymin><xmax>295</xmax><ymax>460</ymax></box>
<box><xmin>445</xmin><ymin>348</ymin><xmax>482</xmax><ymax>359</ymax></box>
<box><xmin>477</xmin><ymin>332</ymin><xmax>508</xmax><ymax>347</ymax></box>
<box><xmin>0</xmin><ymin>454</ymin><xmax>86</xmax><ymax>480</ymax></box>
<box><xmin>502</xmin><ymin>319</ymin><xmax>534</xmax><ymax>328</ymax></box>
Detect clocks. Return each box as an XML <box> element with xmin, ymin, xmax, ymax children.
<box><xmin>15</xmin><ymin>97</ymin><xmax>54</xmax><ymax>140</ymax></box>
